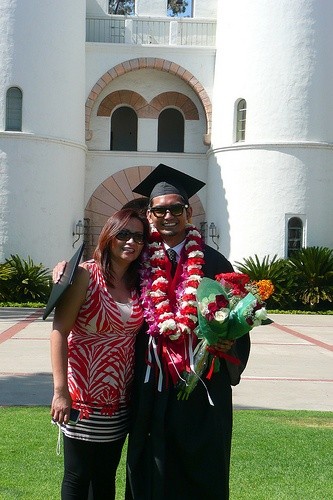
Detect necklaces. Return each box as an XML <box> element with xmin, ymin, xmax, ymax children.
<box><xmin>138</xmin><ymin>221</ymin><xmax>202</xmax><ymax>343</ymax></box>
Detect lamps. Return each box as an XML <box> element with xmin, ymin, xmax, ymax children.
<box><xmin>72</xmin><ymin>219</ymin><xmax>84</xmax><ymax>247</ymax></box>
<box><xmin>209</xmin><ymin>222</ymin><xmax>219</xmax><ymax>250</ymax></box>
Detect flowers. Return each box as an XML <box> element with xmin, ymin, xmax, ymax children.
<box><xmin>176</xmin><ymin>274</ymin><xmax>274</xmax><ymax>400</ymax></box>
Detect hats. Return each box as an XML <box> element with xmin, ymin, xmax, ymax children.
<box><xmin>132</xmin><ymin>163</ymin><xmax>206</xmax><ymax>206</ymax></box>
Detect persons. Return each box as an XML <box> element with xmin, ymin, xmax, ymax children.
<box><xmin>50</xmin><ymin>162</ymin><xmax>252</xmax><ymax>500</ymax></box>
<box><xmin>48</xmin><ymin>206</ymin><xmax>153</xmax><ymax>500</ymax></box>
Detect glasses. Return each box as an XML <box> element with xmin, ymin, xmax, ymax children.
<box><xmin>115</xmin><ymin>230</ymin><xmax>147</xmax><ymax>245</ymax></box>
<box><xmin>150</xmin><ymin>203</ymin><xmax>190</xmax><ymax>218</ymax></box>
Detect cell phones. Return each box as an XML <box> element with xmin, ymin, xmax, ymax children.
<box><xmin>68</xmin><ymin>408</ymin><xmax>80</xmax><ymax>424</ymax></box>
